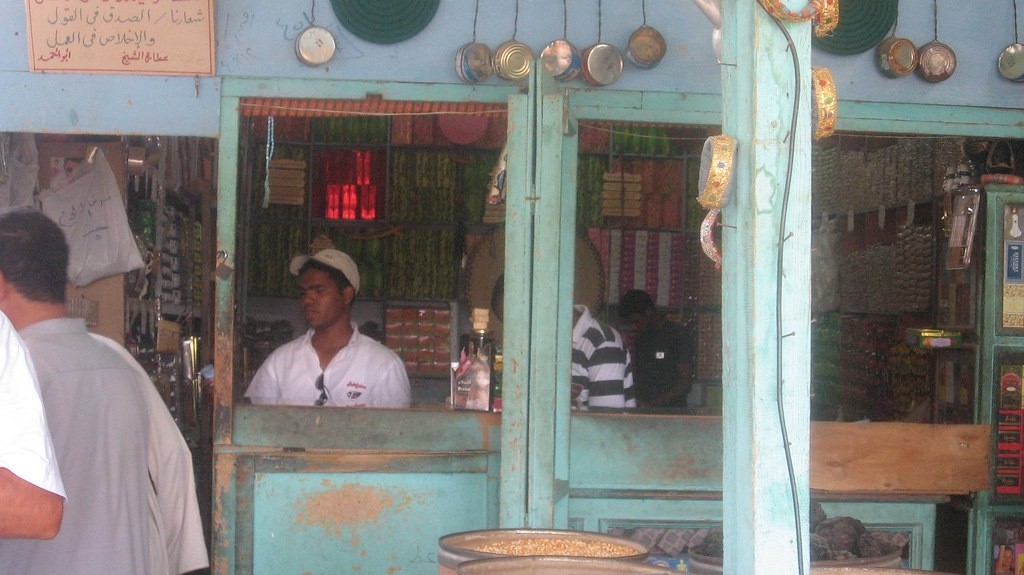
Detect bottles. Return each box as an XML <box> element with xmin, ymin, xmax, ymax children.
<box><xmin>457</xmin><ymin>330</ymin><xmax>488</xmax><ymax>409</ymax></box>
<box><xmin>493</xmin><ymin>348</ymin><xmax>503</xmax><ymax>413</ymax></box>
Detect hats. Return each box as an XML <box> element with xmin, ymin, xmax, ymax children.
<box><xmin>290</xmin><ymin>249</ymin><xmax>360</xmax><ymax>296</ymax></box>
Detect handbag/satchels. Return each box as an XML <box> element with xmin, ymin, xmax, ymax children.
<box><xmin>38</xmin><ymin>145</ymin><xmax>145</xmax><ymax>288</ymax></box>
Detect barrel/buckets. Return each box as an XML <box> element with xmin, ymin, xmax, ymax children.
<box><xmin>438</xmin><ymin>528</ymin><xmax>649</xmax><ymax>575</ymax></box>
<box><xmin>458</xmin><ymin>557</ymin><xmax>690</xmax><ymax>575</ymax></box>
<box><xmin>688</xmin><ymin>547</ymin><xmax>902</xmax><ymax>575</ymax></box>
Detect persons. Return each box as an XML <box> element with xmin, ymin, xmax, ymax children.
<box><xmin>0</xmin><ymin>208</ymin><xmax>209</xmax><ymax>575</ymax></box>
<box><xmin>490</xmin><ymin>273</ymin><xmax>636</xmax><ymax>409</ymax></box>
<box><xmin>622</xmin><ymin>289</ymin><xmax>695</xmax><ymax>410</ymax></box>
<box><xmin>245</xmin><ymin>250</ymin><xmax>412</xmax><ymax>408</ymax></box>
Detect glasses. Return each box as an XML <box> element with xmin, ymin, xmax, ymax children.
<box><xmin>315</xmin><ymin>373</ymin><xmax>331</xmax><ymax>405</ymax></box>
